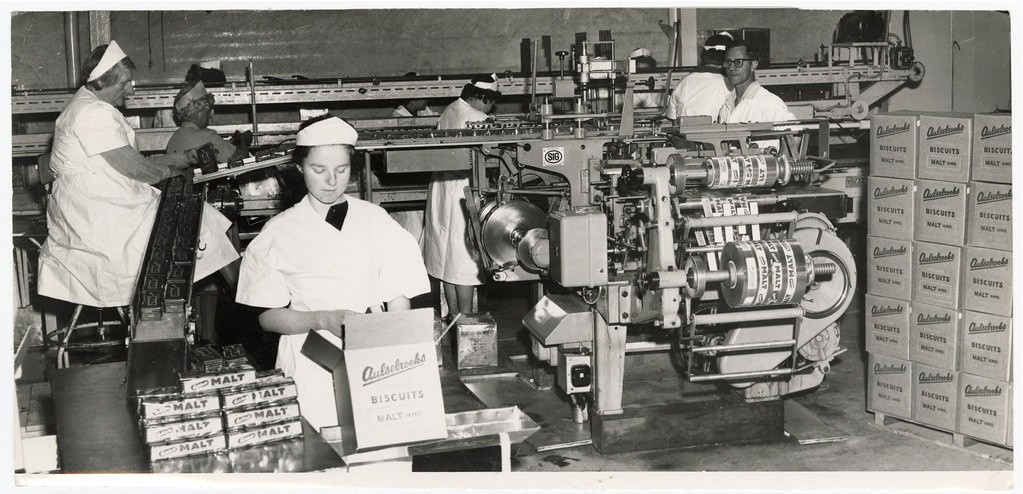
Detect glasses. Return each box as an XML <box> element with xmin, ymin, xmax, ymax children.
<box><xmin>722</xmin><ymin>59</ymin><xmax>754</xmax><ymax>68</ymax></box>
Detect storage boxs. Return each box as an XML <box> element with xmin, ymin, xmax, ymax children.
<box><xmin>909</xmin><ymin>302</ymin><xmax>964</xmax><ymax>373</ymax></box>
<box><xmin>300</xmin><ymin>307</ymin><xmax>460</xmax><ymax>453</ymax></box>
<box><xmin>451</xmin><ymin>313</ymin><xmax>498</xmax><ymax>369</ymax></box>
<box><xmin>972</xmin><ymin>111</ymin><xmax>1012</xmax><ymax>184</ymax></box>
<box><xmin>914</xmin><ymin>179</ymin><xmax>968</xmax><ymax>248</ymax></box>
<box><xmin>866</xmin><ymin>354</ymin><xmax>911</xmax><ymax>420</ymax></box>
<box><xmin>912</xmin><ymin>238</ymin><xmax>963</xmax><ymax>311</ymax></box>
<box><xmin>960</xmin><ymin>308</ymin><xmax>1013</xmax><ymax>384</ymax></box>
<box><xmin>867</xmin><ymin>176</ymin><xmax>916</xmax><ymax>241</ymax></box>
<box><xmin>919</xmin><ymin>112</ymin><xmax>974</xmax><ymax>183</ymax></box>
<box><xmin>912</xmin><ymin>361</ymin><xmax>958</xmax><ymax>431</ymax></box>
<box><xmin>868</xmin><ymin>108</ymin><xmax>928</xmax><ymax>180</ymax></box>
<box><xmin>957</xmin><ymin>371</ymin><xmax>1011</xmax><ymax>444</ymax></box>
<box><xmin>964</xmin><ymin>243</ymin><xmax>1013</xmax><ymax>317</ymax></box>
<box><xmin>967</xmin><ymin>180</ymin><xmax>1012</xmax><ymax>252</ymax></box>
<box><xmin>865</xmin><ymin>294</ymin><xmax>910</xmax><ymax>361</ymax></box>
<box><xmin>866</xmin><ymin>234</ymin><xmax>914</xmax><ymax>301</ymax></box>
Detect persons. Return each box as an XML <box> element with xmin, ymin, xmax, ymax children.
<box><xmin>666</xmin><ymin>35</ymin><xmax>736</xmax><ymax>122</ymax></box>
<box><xmin>418</xmin><ymin>75</ymin><xmax>502</xmax><ymax>315</ymax></box>
<box><xmin>718</xmin><ymin>41</ymin><xmax>799</xmax><ymax>150</ymax></box>
<box><xmin>38</xmin><ymin>41</ymin><xmax>442</xmax><ymax>429</ymax></box>
<box><xmin>614</xmin><ymin>48</ymin><xmax>670</xmax><ymax>108</ymax></box>
